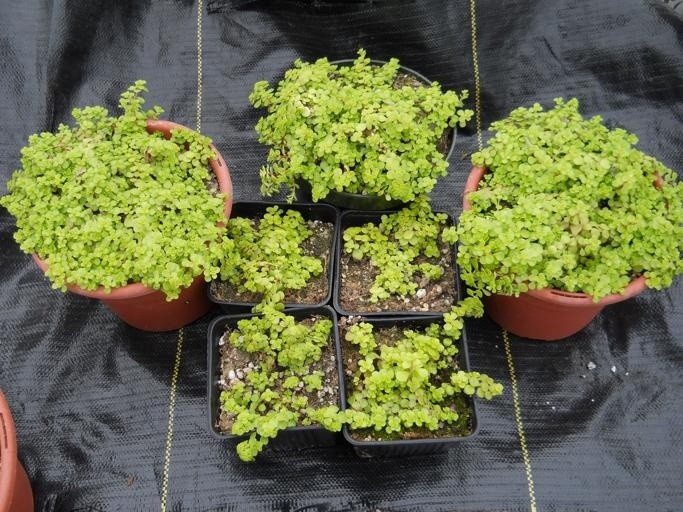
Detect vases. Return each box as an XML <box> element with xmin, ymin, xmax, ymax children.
<box><xmin>0</xmin><ymin>386</ymin><xmax>35</xmax><ymax>511</ymax></box>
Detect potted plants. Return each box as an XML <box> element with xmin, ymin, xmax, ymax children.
<box><xmin>0</xmin><ymin>45</ymin><xmax>682</xmax><ymax>464</ymax></box>
